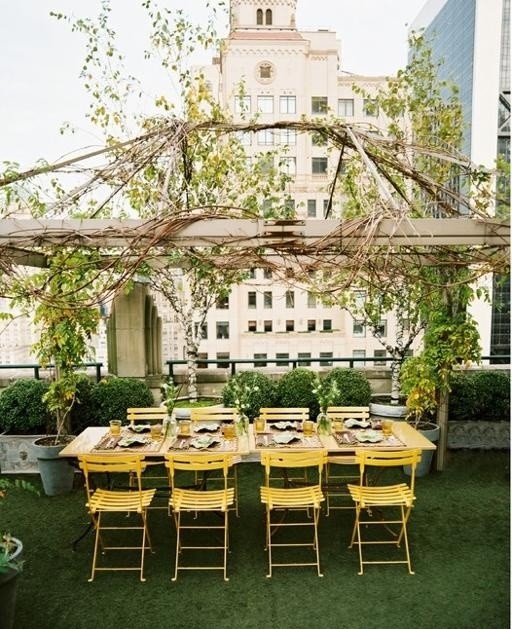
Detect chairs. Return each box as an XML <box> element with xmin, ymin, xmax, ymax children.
<box><xmin>77</xmin><ymin>405</ymin><xmax>434</xmax><ymax>542</ymax></box>
<box><xmin>260</xmin><ymin>450</ymin><xmax>326</xmax><ymax>578</ymax></box>
<box><xmin>346</xmin><ymin>447</ymin><xmax>416</xmax><ymax>575</ymax></box>
<box><xmin>81</xmin><ymin>452</ymin><xmax>157</xmax><ymax>582</ymax></box>
<box><xmin>163</xmin><ymin>452</ymin><xmax>236</xmax><ymax>580</ymax></box>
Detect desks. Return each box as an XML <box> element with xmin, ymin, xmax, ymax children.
<box><xmin>58</xmin><ymin>425</ymin><xmax>440</xmax><ymax>556</ymax></box>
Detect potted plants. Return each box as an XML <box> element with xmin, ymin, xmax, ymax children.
<box><xmin>397</xmin><ymin>309</ymin><xmax>484</xmax><ymax>479</ymax></box>
<box><xmin>24</xmin><ymin>286</ymin><xmax>104</xmax><ymax>496</ymax></box>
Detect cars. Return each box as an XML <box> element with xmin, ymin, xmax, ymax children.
<box><xmin>179</xmin><ymin>420</ymin><xmax>192</xmax><ymax>434</ymax></box>
<box><xmin>222</xmin><ymin>424</ymin><xmax>235</xmax><ymax>440</ymax></box>
<box><xmin>151</xmin><ymin>425</ymin><xmax>162</xmax><ymax>440</ymax></box>
<box><xmin>381</xmin><ymin>420</ymin><xmax>394</xmax><ymax>436</ymax></box>
<box><xmin>254</xmin><ymin>416</ymin><xmax>266</xmax><ymax>432</ymax></box>
<box><xmin>333</xmin><ymin>416</ymin><xmax>344</xmax><ymax>432</ymax></box>
<box><xmin>303</xmin><ymin>421</ymin><xmax>314</xmax><ymax>438</ymax></box>
<box><xmin>109</xmin><ymin>419</ymin><xmax>122</xmax><ymax>435</ymax></box>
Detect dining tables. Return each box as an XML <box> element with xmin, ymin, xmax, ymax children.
<box><xmin>397</xmin><ymin>309</ymin><xmax>484</xmax><ymax>479</ymax></box>
<box><xmin>24</xmin><ymin>286</ymin><xmax>104</xmax><ymax>496</ymax></box>
